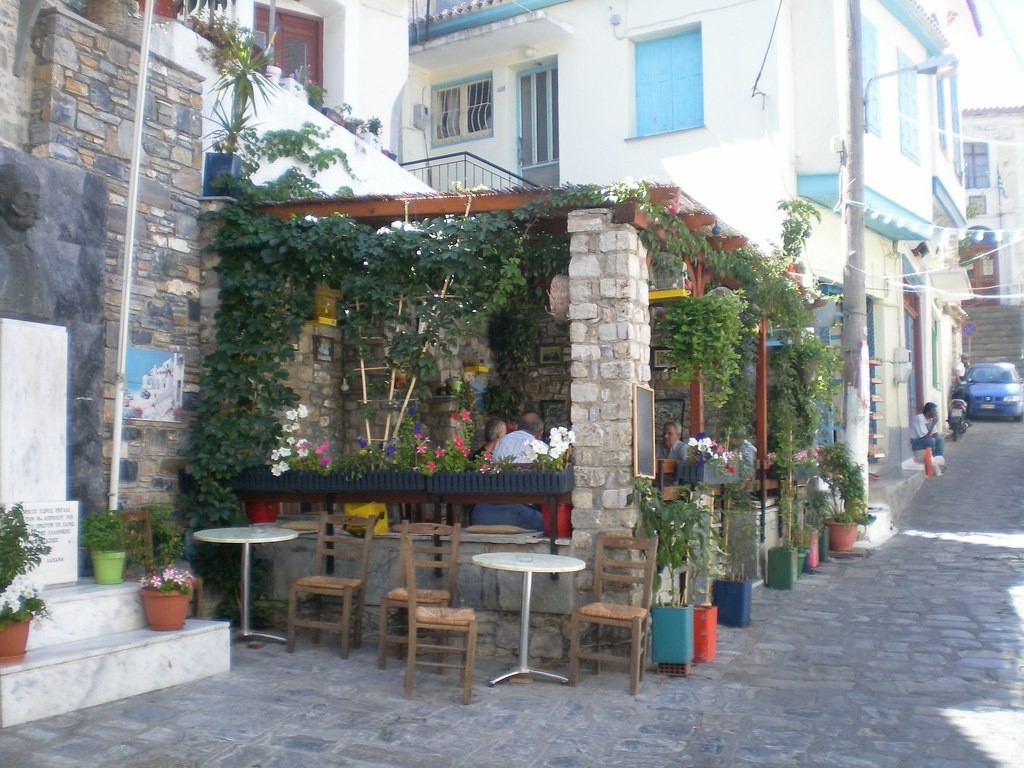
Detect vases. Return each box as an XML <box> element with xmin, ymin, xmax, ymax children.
<box><xmin>427</xmin><ymin>468</ymin><xmax>576</xmax><ymax>495</ymax></box>
<box><xmin>281</xmin><ymin>77</ymin><xmax>303</xmax><ymax>97</ymax></box>
<box><xmin>768</xmin><ymin>545</ymin><xmax>796</xmax><ymax>590</ymax></box>
<box><xmin>241</xmin><ymin>469</ymin><xmax>318</xmax><ymax>493</ymax></box>
<box><xmin>140</xmin><ymin>584</ymin><xmax>189</xmax><ymax>630</ymax></box>
<box><xmin>0</xmin><ymin>613</ymin><xmax>33</xmax><ymax>662</ymax></box>
<box><xmin>319</xmin><ymin>471</ymin><xmax>425</xmax><ymax>493</ymax></box>
<box><xmin>678</xmin><ymin>462</ymin><xmax>741</xmax><ymax>485</ymax></box>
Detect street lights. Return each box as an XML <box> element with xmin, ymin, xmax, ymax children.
<box><xmin>830</xmin><ymin>51</ymin><xmax>962</xmax><ymax>514</ymax></box>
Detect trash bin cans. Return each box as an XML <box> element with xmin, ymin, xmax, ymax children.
<box><xmin>693</xmin><ymin>604</ymin><xmax>717</xmax><ymax>663</ymax></box>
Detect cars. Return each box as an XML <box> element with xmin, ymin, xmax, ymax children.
<box><xmin>960</xmin><ymin>362</ymin><xmax>1024</xmax><ymax>422</ymax></box>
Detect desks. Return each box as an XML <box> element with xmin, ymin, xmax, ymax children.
<box><xmin>471</xmin><ymin>552</ymin><xmax>587</xmax><ymax>687</ymax></box>
<box><xmin>192</xmin><ymin>526</ymin><xmax>299</xmax><ymax>644</ymax></box>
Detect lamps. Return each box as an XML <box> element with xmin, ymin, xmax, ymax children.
<box><xmin>524</xmin><ymin>47</ymin><xmax>537</xmax><ymax>57</ymax></box>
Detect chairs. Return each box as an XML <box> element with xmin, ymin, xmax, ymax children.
<box><xmin>402</xmin><ymin>535</ymin><xmax>479</xmax><ymax>706</ymax></box>
<box><xmin>570</xmin><ymin>531</ymin><xmax>659</xmax><ymax>697</ymax></box>
<box><xmin>379</xmin><ymin>519</ymin><xmax>462</xmax><ymax>675</ymax></box>
<box><xmin>115</xmin><ymin>506</ymin><xmax>204</xmax><ymax>619</ymax></box>
<box><xmin>286</xmin><ymin>511</ymin><xmax>376</xmax><ymax>660</ymax></box>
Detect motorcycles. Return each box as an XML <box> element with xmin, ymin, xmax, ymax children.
<box><xmin>944</xmin><ymin>377</ymin><xmax>976</xmax><ymax>442</ymax></box>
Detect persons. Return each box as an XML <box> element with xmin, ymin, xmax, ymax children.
<box><xmin>956</xmin><ymin>353</ymin><xmax>970</xmax><ymax>384</ymax></box>
<box><xmin>471</xmin><ymin>418</ymin><xmax>506</xmax><ymax>464</ymax></box>
<box><xmin>910</xmin><ymin>402</ymin><xmax>944</xmax><ymax>457</ymax></box>
<box><xmin>655</xmin><ymin>420</ymin><xmax>688</xmax><ymax>461</ymax></box>
<box><xmin>472</xmin><ymin>410</ymin><xmax>544</xmax><ymax>532</ymax></box>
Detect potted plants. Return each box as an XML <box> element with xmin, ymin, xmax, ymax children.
<box><xmin>790</xmin><ymin>443</ymin><xmax>869</xmax><ymax>575</ymax></box>
<box><xmin>632</xmin><ymin>476</ymin><xmax>703</xmax><ymax>676</ymax></box>
<box><xmin>670</xmin><ymin>485</ymin><xmax>718</xmax><ymax>664</ymax></box>
<box><xmin>356</xmin><ymin>116</ymin><xmax>382</xmax><ymax>152</ymax></box>
<box><xmin>309</xmin><ymin>97</ymin><xmax>324</xmax><ymax>113</ymax></box>
<box><xmin>204</xmin><ymin>39</ymin><xmax>285</xmax><ymax>198</ymax></box>
<box><xmin>707</xmin><ymin>490</ymin><xmax>765</xmax><ymax>628</ymax></box>
<box><xmin>323</xmin><ymin>103</ymin><xmax>351</xmax><ymax>128</ymax></box>
<box><xmin>382</xmin><ymin>148</ymin><xmax>401</xmax><ymax>162</ymax></box>
<box><xmin>265</xmin><ymin>45</ymin><xmax>283</xmax><ymax>84</ymax></box>
<box><xmin>84</xmin><ymin>509</ymin><xmax>132</xmax><ymax>588</ymax></box>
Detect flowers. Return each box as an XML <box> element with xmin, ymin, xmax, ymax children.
<box><xmin>288</xmin><ymin>62</ymin><xmax>327</xmax><ymax>103</ymax></box>
<box><xmin>141</xmin><ymin>568</ymin><xmax>192</xmax><ymax>589</ymax></box>
<box><xmin>765</xmin><ymin>450</ymin><xmax>807</xmax><ymax>548</ymax></box>
<box><xmin>0</xmin><ymin>573</ymin><xmax>47</xmax><ymax>622</ymax></box>
<box><xmin>684</xmin><ymin>431</ymin><xmax>736</xmax><ymax>478</ymax></box>
<box><xmin>272</xmin><ymin>403</ymin><xmax>577</xmax><ymax>477</ymax></box>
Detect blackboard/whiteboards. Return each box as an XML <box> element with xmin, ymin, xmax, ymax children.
<box><xmin>632</xmin><ymin>383</ymin><xmax>656</xmax><ymax>480</ymax></box>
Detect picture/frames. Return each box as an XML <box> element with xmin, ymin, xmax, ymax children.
<box><xmin>632</xmin><ymin>383</ymin><xmax>657</xmax><ymax>480</ymax></box>
<box><xmin>311</xmin><ymin>335</ymin><xmax>334</xmax><ymax>363</ymax></box>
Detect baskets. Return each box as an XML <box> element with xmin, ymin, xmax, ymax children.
<box><xmin>545</xmin><ymin>274</ymin><xmax>570</xmax><ymax>325</ymax></box>
